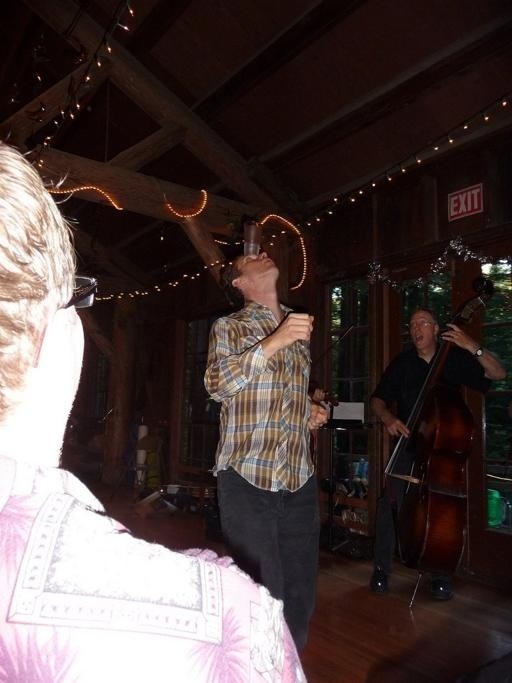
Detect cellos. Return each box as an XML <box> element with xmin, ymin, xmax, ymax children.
<box><xmin>393</xmin><ymin>297</ymin><xmax>485</xmax><ymax>575</ymax></box>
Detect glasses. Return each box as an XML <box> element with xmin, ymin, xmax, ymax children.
<box><xmin>410</xmin><ymin>320</ymin><xmax>433</xmax><ymax>328</ymax></box>
<box><xmin>61</xmin><ymin>276</ymin><xmax>99</xmax><ymax>310</ymax></box>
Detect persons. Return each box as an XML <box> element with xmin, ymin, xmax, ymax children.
<box><xmin>0</xmin><ymin>140</ymin><xmax>307</xmax><ymax>683</ymax></box>
<box><xmin>368</xmin><ymin>308</ymin><xmax>508</xmax><ymax>601</ymax></box>
<box><xmin>203</xmin><ymin>252</ymin><xmax>329</xmax><ymax>652</ymax></box>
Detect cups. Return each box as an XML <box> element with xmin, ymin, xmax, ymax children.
<box><xmin>242</xmin><ymin>222</ymin><xmax>263</xmax><ymax>255</ymax></box>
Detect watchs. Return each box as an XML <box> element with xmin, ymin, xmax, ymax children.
<box><xmin>472</xmin><ymin>346</ymin><xmax>483</xmax><ymax>356</ymax></box>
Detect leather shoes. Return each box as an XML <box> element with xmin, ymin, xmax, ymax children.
<box><xmin>368</xmin><ymin>570</ymin><xmax>388</xmax><ymax>594</ymax></box>
<box><xmin>430</xmin><ymin>579</ymin><xmax>455</xmax><ymax>600</ymax></box>
